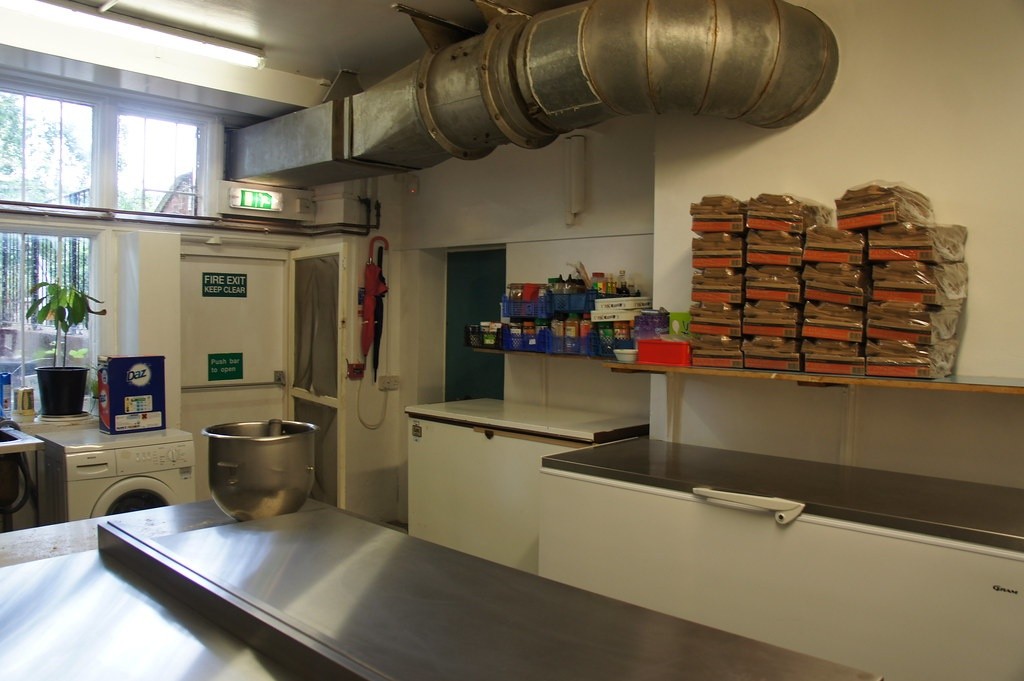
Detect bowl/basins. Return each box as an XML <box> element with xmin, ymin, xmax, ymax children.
<box><xmin>202</xmin><ymin>421</ymin><xmax>318</xmax><ymax>524</ymax></box>
<box><xmin>613</xmin><ymin>349</ymin><xmax>637</xmax><ymax>363</ymax></box>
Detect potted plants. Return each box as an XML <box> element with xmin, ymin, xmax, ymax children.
<box><xmin>25</xmin><ymin>281</ymin><xmax>107</xmax><ymax>415</ymax></box>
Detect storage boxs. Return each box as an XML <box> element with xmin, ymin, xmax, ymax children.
<box><xmin>96</xmin><ymin>354</ymin><xmax>166</xmax><ymax>435</ymax></box>
<box><xmin>462</xmin><ymin>180</ymin><xmax>970</xmax><ymax>380</ymax></box>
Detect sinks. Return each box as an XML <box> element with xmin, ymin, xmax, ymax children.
<box><xmin>0</xmin><ymin>428</ymin><xmax>44</xmax><ymax>454</ymax></box>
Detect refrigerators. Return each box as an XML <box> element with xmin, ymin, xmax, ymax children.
<box><xmin>539</xmin><ymin>435</ymin><xmax>1023</xmax><ymax>681</ymax></box>
<box><xmin>406</xmin><ymin>396</ymin><xmax>650</xmax><ymax>577</ymax></box>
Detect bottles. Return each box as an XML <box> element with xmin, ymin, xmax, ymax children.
<box><xmin>509</xmin><ymin>312</ymin><xmax>635</xmax><ymax>354</ymax></box>
<box><xmin>591</xmin><ymin>268</ymin><xmax>637</xmax><ymax>297</ymax></box>
<box><xmin>0</xmin><ymin>372</ymin><xmax>11</xmax><ymax>419</ymax></box>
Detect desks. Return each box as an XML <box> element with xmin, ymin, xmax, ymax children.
<box><xmin>0</xmin><ymin>497</ymin><xmax>886</xmax><ymax>680</ymax></box>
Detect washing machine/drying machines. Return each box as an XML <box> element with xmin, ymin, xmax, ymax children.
<box><xmin>35</xmin><ymin>426</ymin><xmax>196</xmax><ymax>527</ymax></box>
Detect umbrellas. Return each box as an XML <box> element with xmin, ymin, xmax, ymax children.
<box><xmin>361</xmin><ymin>236</ymin><xmax>389</xmax><ymax>370</ymax></box>
<box><xmin>372</xmin><ymin>246</ymin><xmax>386</xmax><ymax>383</ymax></box>
